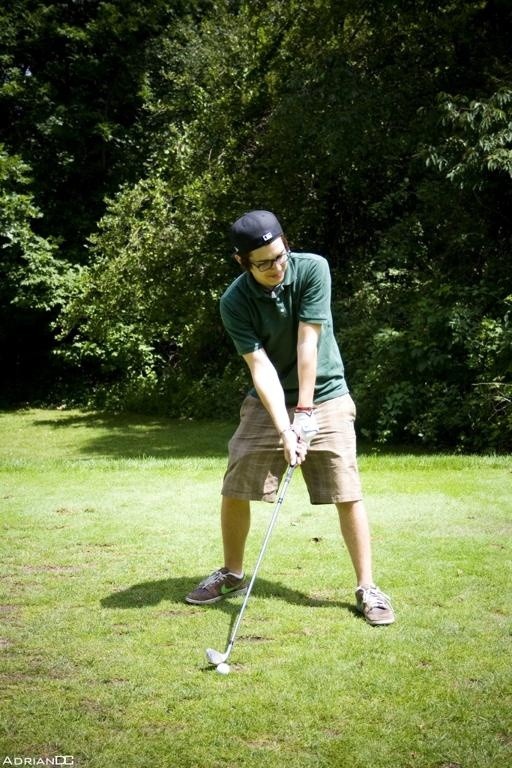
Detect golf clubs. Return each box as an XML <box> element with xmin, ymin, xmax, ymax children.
<box><xmin>206</xmin><ymin>463</ymin><xmax>299</xmax><ymax>665</ymax></box>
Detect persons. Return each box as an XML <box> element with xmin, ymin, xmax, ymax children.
<box><xmin>183</xmin><ymin>210</ymin><xmax>396</xmax><ymax>624</ymax></box>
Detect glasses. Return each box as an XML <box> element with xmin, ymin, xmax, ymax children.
<box><xmin>251</xmin><ymin>252</ymin><xmax>291</xmax><ymax>271</ymax></box>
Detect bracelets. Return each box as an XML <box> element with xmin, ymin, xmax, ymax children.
<box><xmin>295</xmin><ymin>406</ymin><xmax>317</xmax><ymax>411</ymax></box>
<box><xmin>279</xmin><ymin>427</ymin><xmax>291</xmax><ymax>436</ymax></box>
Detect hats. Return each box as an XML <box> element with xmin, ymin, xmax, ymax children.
<box><xmin>231</xmin><ymin>209</ymin><xmax>283</xmax><ymax>253</ymax></box>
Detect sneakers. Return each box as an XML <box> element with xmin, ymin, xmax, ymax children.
<box><xmin>185</xmin><ymin>567</ymin><xmax>247</xmax><ymax>604</ymax></box>
<box><xmin>356</xmin><ymin>584</ymin><xmax>395</xmax><ymax>624</ymax></box>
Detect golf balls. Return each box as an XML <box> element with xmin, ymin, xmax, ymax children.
<box><xmin>216</xmin><ymin>663</ymin><xmax>231</xmax><ymax>677</ymax></box>
